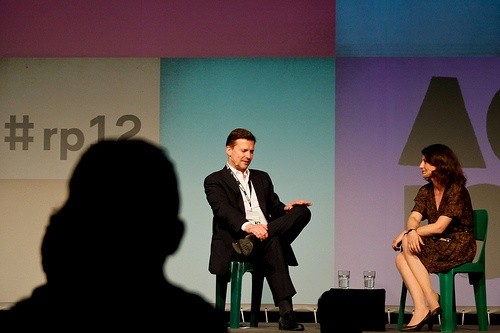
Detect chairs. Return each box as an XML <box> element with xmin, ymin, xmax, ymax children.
<box><xmin>397</xmin><ymin>209</ymin><xmax>490</xmax><ymax>333</ymax></box>
<box><xmin>215</xmin><ymin>261</ymin><xmax>265</xmax><ymax>329</ymax></box>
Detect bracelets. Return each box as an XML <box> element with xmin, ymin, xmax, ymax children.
<box><xmin>405</xmin><ymin>228</ymin><xmax>415</xmax><ymax>234</ymax></box>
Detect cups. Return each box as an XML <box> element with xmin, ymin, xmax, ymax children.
<box><xmin>364</xmin><ymin>271</ymin><xmax>376</xmax><ymax>288</ymax></box>
<box><xmin>338</xmin><ymin>270</ymin><xmax>350</xmax><ymax>288</ymax></box>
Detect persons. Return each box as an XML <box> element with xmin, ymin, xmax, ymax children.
<box><xmin>203</xmin><ymin>127</ymin><xmax>313</xmax><ymax>332</ymax></box>
<box><xmin>391</xmin><ymin>144</ymin><xmax>478</xmax><ymax>333</ymax></box>
<box><xmin>0</xmin><ymin>136</ymin><xmax>228</xmax><ymax>333</ymax></box>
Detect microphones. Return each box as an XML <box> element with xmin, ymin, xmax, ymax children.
<box><xmin>231</xmin><ymin>158</ymin><xmax>238</xmax><ymax>166</ymax></box>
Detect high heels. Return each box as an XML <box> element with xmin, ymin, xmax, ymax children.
<box><xmin>430</xmin><ymin>293</ymin><xmax>442</xmax><ymax>316</ymax></box>
<box><xmin>400</xmin><ymin>310</ymin><xmax>433</xmax><ymax>332</ymax></box>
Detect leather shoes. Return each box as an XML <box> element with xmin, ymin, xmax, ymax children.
<box><xmin>232</xmin><ymin>239</ymin><xmax>255</xmax><ymax>258</ymax></box>
<box><xmin>278</xmin><ymin>311</ymin><xmax>305</xmax><ymax>331</ymax></box>
